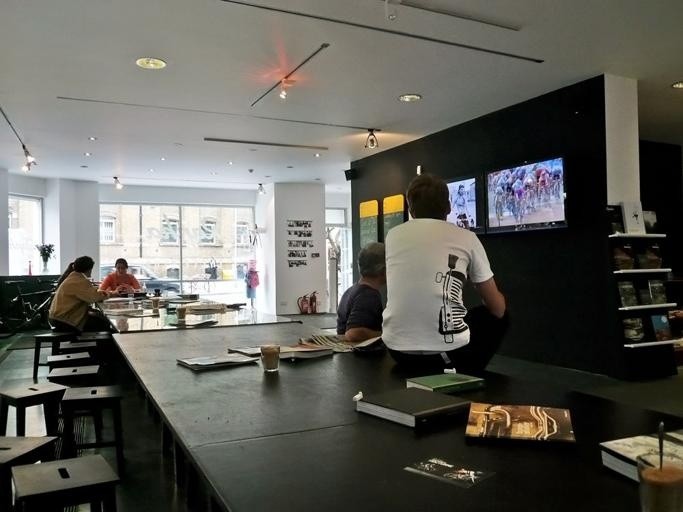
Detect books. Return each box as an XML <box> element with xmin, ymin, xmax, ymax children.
<box><xmin>104</xmin><ymin>308</ymin><xmax>144</xmax><ymax>316</ymax></box>
<box><xmin>178</xmin><ymin>294</ymin><xmax>199</xmax><ymax>301</ymax></box>
<box><xmin>623</xmin><ymin>318</ymin><xmax>645</xmax><ymax>344</ymax></box>
<box><xmin>597</xmin><ymin>428</ymin><xmax>682</xmax><ymax>485</ymax></box>
<box><xmin>613</xmin><ymin>242</ymin><xmax>664</xmax><ymax>270</ymax></box>
<box><xmin>605</xmin><ymin>201</ymin><xmax>660</xmax><ymax>235</ymax></box>
<box><xmin>175</xmin><ymin>352</ymin><xmax>259</xmax><ymax>371</ymax></box>
<box><xmin>227</xmin><ymin>345</ymin><xmax>263</xmax><ymax>357</ymax></box>
<box><xmin>279</xmin><ymin>343</ymin><xmax>333</xmax><ymax>360</ymax></box>
<box><xmin>464</xmin><ymin>402</ymin><xmax>579</xmax><ymax>444</ymax></box>
<box><xmin>650</xmin><ymin>314</ymin><xmax>673</xmax><ymax>342</ymax></box>
<box><xmin>405</xmin><ymin>372</ymin><xmax>486</xmax><ymax>395</ymax></box>
<box><xmin>619</xmin><ymin>279</ymin><xmax>668</xmax><ymax>307</ymax></box>
<box><xmin>354</xmin><ymin>385</ymin><xmax>471</xmax><ymax>428</ymax></box>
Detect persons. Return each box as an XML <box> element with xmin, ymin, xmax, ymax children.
<box><xmin>380</xmin><ymin>174</ymin><xmax>513</xmax><ymax>372</ymax></box>
<box><xmin>488</xmin><ymin>159</ymin><xmax>564</xmax><ymax>226</ymax></box>
<box><xmin>337</xmin><ymin>243</ymin><xmax>386</xmax><ymax>341</ymax></box>
<box><xmin>48</xmin><ymin>256</ymin><xmax>109</xmax><ymax>333</ymax></box>
<box><xmin>244</xmin><ymin>259</ymin><xmax>259</xmax><ymax>300</ymax></box>
<box><xmin>98</xmin><ymin>259</ymin><xmax>144</xmax><ymax>294</ymax></box>
<box><xmin>452</xmin><ymin>184</ymin><xmax>475</xmax><ymax>226</ymax></box>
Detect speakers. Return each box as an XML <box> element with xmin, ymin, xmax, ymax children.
<box><xmin>344</xmin><ymin>168</ymin><xmax>360</xmax><ymax>181</ymax></box>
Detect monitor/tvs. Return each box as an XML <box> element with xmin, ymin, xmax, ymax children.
<box><xmin>485</xmin><ymin>151</ymin><xmax>570</xmax><ymax>234</ymax></box>
<box><xmin>407</xmin><ymin>170</ymin><xmax>486</xmax><ymax>235</ymax></box>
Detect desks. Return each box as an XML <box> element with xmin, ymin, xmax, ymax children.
<box><xmin>97</xmin><ymin>301</ymin><xmax>302</xmax><ymax>332</ymax></box>
<box><xmin>190</xmin><ymin>392</ymin><xmax>683</xmax><ymax>511</ymax></box>
<box><xmin>111</xmin><ymin>321</ymin><xmax>571</xmax><ymax>488</ymax></box>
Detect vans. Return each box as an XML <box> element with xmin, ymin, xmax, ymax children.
<box><xmin>100</xmin><ymin>265</ymin><xmax>184</xmax><ymax>294</ymax></box>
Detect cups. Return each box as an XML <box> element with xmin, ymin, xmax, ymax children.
<box><xmin>637</xmin><ymin>452</ymin><xmax>682</xmax><ymax>511</ymax></box>
<box><xmin>128</xmin><ymin>293</ymin><xmax>134</xmax><ymax>298</ymax></box>
<box><xmin>152</xmin><ymin>297</ymin><xmax>160</xmax><ymax>309</ymax></box>
<box><xmin>176</xmin><ymin>305</ymin><xmax>187</xmax><ymax>320</ymax></box>
<box><xmin>260</xmin><ymin>344</ymin><xmax>280</xmax><ymax>373</ymax></box>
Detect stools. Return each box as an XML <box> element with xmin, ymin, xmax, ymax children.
<box><xmin>0</xmin><ymin>382</ymin><xmax>70</xmax><ymax>437</ymax></box>
<box><xmin>47</xmin><ymin>352</ymin><xmax>90</xmax><ymax>374</ymax></box>
<box><xmin>77</xmin><ymin>335</ymin><xmax>110</xmax><ymax>340</ymax></box>
<box><xmin>11</xmin><ymin>454</ymin><xmax>120</xmax><ymax>512</ymax></box>
<box><xmin>33</xmin><ymin>332</ymin><xmax>72</xmax><ymax>384</ymax></box>
<box><xmin>47</xmin><ymin>364</ymin><xmax>103</xmax><ymax>427</ymax></box>
<box><xmin>0</xmin><ymin>437</ymin><xmax>58</xmax><ymax>482</ymax></box>
<box><xmin>81</xmin><ymin>331</ymin><xmax>110</xmax><ymax>335</ymax></box>
<box><xmin>60</xmin><ymin>341</ymin><xmax>98</xmax><ymax>353</ymax></box>
<box><xmin>62</xmin><ymin>385</ymin><xmax>126</xmax><ymax>474</ymax></box>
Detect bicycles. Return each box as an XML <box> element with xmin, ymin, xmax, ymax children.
<box><xmin>490</xmin><ymin>169</ymin><xmax>561</xmax><ymax>225</ymax></box>
<box><xmin>0</xmin><ymin>278</ymin><xmax>57</xmax><ymax>339</ymax></box>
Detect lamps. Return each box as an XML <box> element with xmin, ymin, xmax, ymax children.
<box><xmin>22</xmin><ymin>149</ymin><xmax>39</xmax><ymax>172</ymax></box>
<box><xmin>279</xmin><ymin>77</ymin><xmax>295</xmax><ymax>101</ymax></box>
<box><xmin>365</xmin><ymin>128</ymin><xmax>378</xmax><ymax>149</ymax></box>
<box><xmin>256</xmin><ymin>183</ymin><xmax>266</xmax><ymax>195</ymax></box>
<box><xmin>114</xmin><ymin>177</ymin><xmax>124</xmax><ymax>191</ymax></box>
